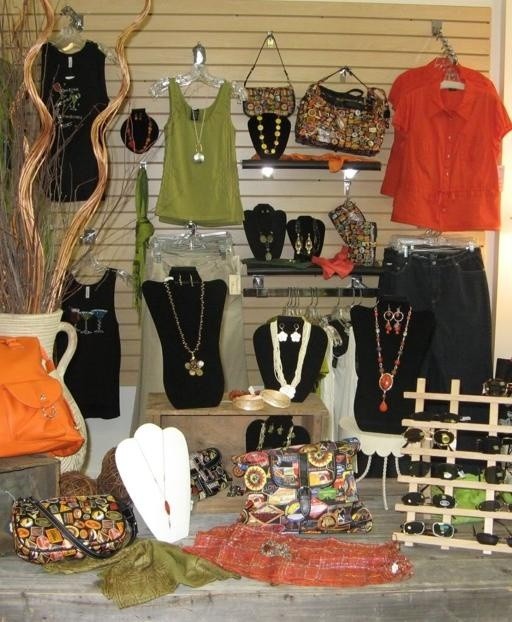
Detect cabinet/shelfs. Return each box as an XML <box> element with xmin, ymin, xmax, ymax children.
<box><xmin>242</xmin><ymin>157</ymin><xmax>394</xmax><ymax>275</ymax></box>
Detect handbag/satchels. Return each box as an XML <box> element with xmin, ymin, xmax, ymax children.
<box><xmin>328</xmin><ymin>186</ymin><xmax>377</xmax><ymax>267</ymax></box>
<box><xmin>242</xmin><ymin>34</ymin><xmax>295</xmax><ymax>117</ymax></box>
<box><xmin>9</xmin><ymin>494</ymin><xmax>137</xmax><ymax>564</ymax></box>
<box><xmin>237</xmin><ymin>438</ymin><xmax>374</xmax><ymax>536</ymax></box>
<box><xmin>0</xmin><ymin>335</ymin><xmax>85</xmax><ymax>458</ymax></box>
<box><xmin>295</xmin><ymin>67</ymin><xmax>390</xmax><ymax>156</ymax></box>
<box><xmin>189</xmin><ymin>448</ymin><xmax>232</xmax><ymax>503</ymax></box>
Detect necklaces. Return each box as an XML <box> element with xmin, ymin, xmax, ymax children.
<box><xmin>257</xmin><ymin>422</ymin><xmax>295</xmax><ymax>452</ymax></box>
<box><xmin>163</xmin><ymin>278</ymin><xmax>210</xmax><ymax>377</ymax></box>
<box><xmin>135</xmin><ymin>430</ymin><xmax>170</xmax><ymax>516</ymax></box>
<box><xmin>258</xmin><ymin>112</ymin><xmax>281</xmax><ymax>155</ymax></box>
<box><xmin>371</xmin><ymin>305</ymin><xmax>413</xmax><ymax>411</ymax></box>
<box><xmin>254</xmin><ymin>212</ymin><xmax>274</xmax><ymax>262</ymax></box>
<box><xmin>191</xmin><ymin>83</ymin><xmax>210</xmax><ymax>164</ymax></box>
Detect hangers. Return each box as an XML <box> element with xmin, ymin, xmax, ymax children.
<box><xmin>153</xmin><ymin>225</ymin><xmax>230</xmax><ymax>258</ymax></box>
<box><xmin>433</xmin><ymin>33</ymin><xmax>466</xmax><ymax>91</ymax></box>
<box><xmin>393</xmin><ymin>227</ymin><xmax>474</xmax><ymax>257</ymax></box>
<box><xmin>153</xmin><ymin>45</ymin><xmax>247</xmax><ymax>101</ymax></box>
<box><xmin>70</xmin><ymin>231</ymin><xmax>131</xmax><ymax>284</ymax></box>
<box><xmin>50</xmin><ymin>7</ymin><xmax>115</xmax><ymax>59</ymax></box>
<box><xmin>284</xmin><ymin>287</ymin><xmax>364</xmax><ymax>325</ymax></box>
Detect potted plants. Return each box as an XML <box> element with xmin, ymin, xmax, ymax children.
<box><xmin>0</xmin><ymin>0</ymin><xmax>178</xmax><ymax>474</ymax></box>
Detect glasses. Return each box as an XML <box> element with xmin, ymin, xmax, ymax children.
<box><xmin>399</xmin><ymin>411</ymin><xmax>463</xmax><ymax>538</ymax></box>
<box><xmin>473</xmin><ymin>435</ymin><xmax>512</xmax><ymax>547</ymax></box>
<box><xmin>481</xmin><ymin>377</ymin><xmax>512</xmax><ymax>396</ymax></box>
